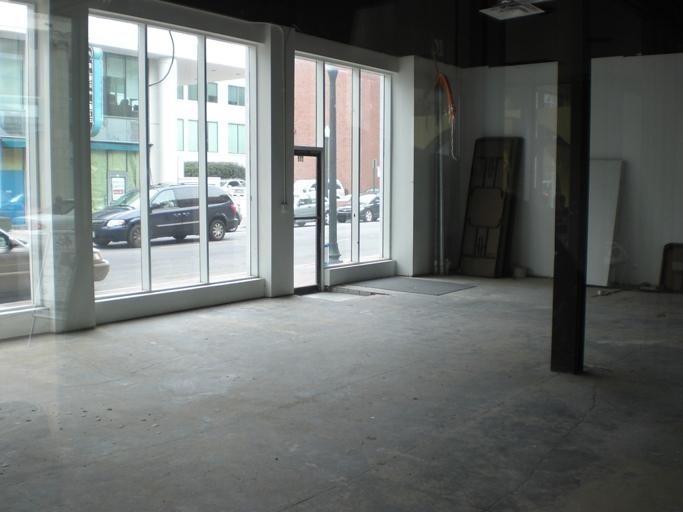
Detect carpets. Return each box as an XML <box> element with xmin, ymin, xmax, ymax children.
<box><xmin>345</xmin><ymin>275</ymin><xmax>478</xmax><ymax>296</ymax></box>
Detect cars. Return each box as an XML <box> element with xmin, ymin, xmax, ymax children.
<box><xmin>293</xmin><ymin>178</ymin><xmax>380</xmax><ymax>226</ymax></box>
<box><xmin>0</xmin><ymin>229</ymin><xmax>110</xmax><ymax>294</ymax></box>
<box><xmin>92</xmin><ymin>181</ymin><xmax>242</xmax><ymax>248</ymax></box>
<box><xmin>216</xmin><ymin>180</ymin><xmax>246</xmax><ymax>196</ymax></box>
